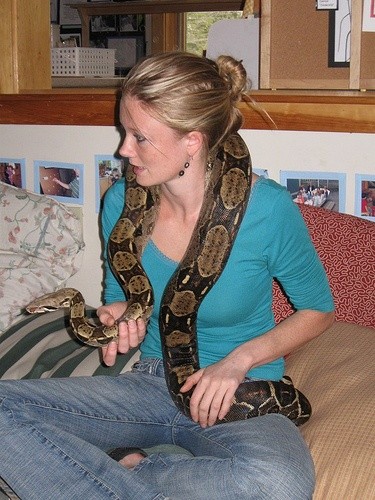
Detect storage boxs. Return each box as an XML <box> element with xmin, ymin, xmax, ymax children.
<box><xmin>49</xmin><ymin>47</ymin><xmax>116</xmax><ymax>78</ymax></box>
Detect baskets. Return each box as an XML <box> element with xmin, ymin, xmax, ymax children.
<box><xmin>50</xmin><ymin>47</ymin><xmax>116</xmax><ymax>77</ymax></box>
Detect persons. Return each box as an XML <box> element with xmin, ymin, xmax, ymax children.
<box><xmin>52</xmin><ymin>168</ymin><xmax>79</xmax><ymax>199</ymax></box>
<box><xmin>0</xmin><ymin>45</ymin><xmax>334</xmax><ymax>500</ymax></box>
